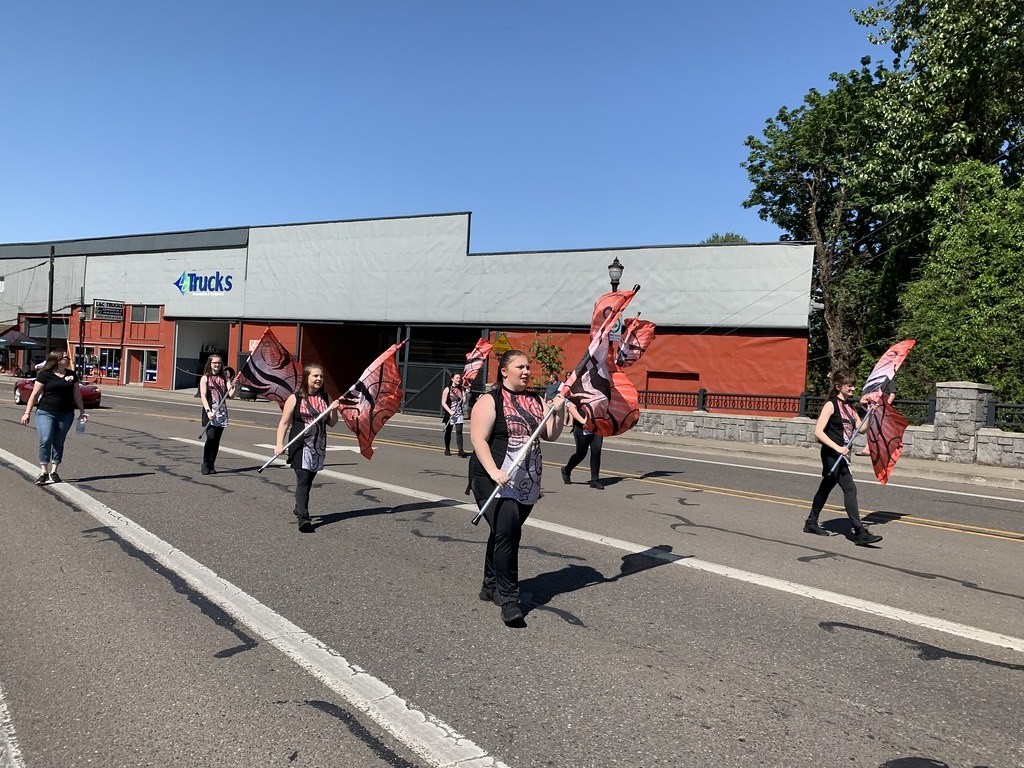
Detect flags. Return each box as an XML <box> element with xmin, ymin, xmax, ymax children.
<box><xmin>559</xmin><ymin>289</ymin><xmax>657</xmax><ymax>437</ymax></box>
<box><xmin>237</xmin><ymin>328</ymin><xmax>307</xmax><ymax>412</ymax></box>
<box><xmin>339</xmin><ymin>344</ymin><xmax>404</xmax><ymax>460</ymax></box>
<box><xmin>860</xmin><ymin>340</ymin><xmax>908</xmax><ymax>486</ymax></box>
<box><xmin>461</xmin><ymin>337</ymin><xmax>494</xmax><ymax>386</ymax></box>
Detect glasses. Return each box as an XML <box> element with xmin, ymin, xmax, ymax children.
<box><xmin>211</xmin><ymin>362</ymin><xmax>223</xmax><ymax>365</ymax></box>
<box><xmin>62</xmin><ymin>356</ymin><xmax>70</xmax><ymax>360</ymax></box>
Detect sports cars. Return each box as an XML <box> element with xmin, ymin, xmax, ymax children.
<box><xmin>13</xmin><ymin>379</ymin><xmax>102</xmax><ymax>408</ymax></box>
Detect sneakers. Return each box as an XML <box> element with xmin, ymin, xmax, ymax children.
<box><xmin>479</xmin><ymin>588</ymin><xmax>500</xmax><ymax>605</ymax></box>
<box><xmin>35</xmin><ymin>471</ymin><xmax>49</xmax><ymax>484</ymax></box>
<box><xmin>50</xmin><ymin>472</ymin><xmax>61</xmax><ymax>483</ymax></box>
<box><xmin>500</xmin><ymin>602</ymin><xmax>524</xmax><ymax>623</ymax></box>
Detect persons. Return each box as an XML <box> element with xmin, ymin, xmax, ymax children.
<box><xmin>853</xmin><ymin>378</ymin><xmax>896</xmax><ymax>457</ymax></box>
<box><xmin>561</xmin><ymin>397</ymin><xmax>605</xmax><ymax>490</ymax></box>
<box><xmin>544</xmin><ymin>374</ymin><xmax>561</xmax><ymax>404</ymax></box>
<box><xmin>562</xmin><ymin>371</ymin><xmax>574</xmax><ymax>427</ymax></box>
<box><xmin>464</xmin><ymin>350</ymin><xmax>566</xmax><ymax>625</ymax></box>
<box><xmin>199</xmin><ymin>355</ymin><xmax>239</xmax><ymax>475</ymax></box>
<box><xmin>441</xmin><ymin>372</ymin><xmax>467</xmax><ymax>456</ymax></box>
<box><xmin>21</xmin><ymin>351</ymin><xmax>89</xmax><ymax>485</ymax></box>
<box><xmin>803</xmin><ymin>367</ymin><xmax>883</xmax><ymax>545</ymax></box>
<box><xmin>273</xmin><ymin>366</ymin><xmax>338</xmax><ymax>528</ymax></box>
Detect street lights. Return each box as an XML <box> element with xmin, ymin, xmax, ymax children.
<box><xmin>77</xmin><ymin>307</ymin><xmax>87</xmax><ymax>376</ymax></box>
<box><xmin>608</xmin><ymin>256</ymin><xmax>625</xmax><ymax>353</ymax></box>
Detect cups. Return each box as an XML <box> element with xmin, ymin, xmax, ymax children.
<box><xmin>76</xmin><ymin>418</ymin><xmax>86</xmax><ymax>434</ymax></box>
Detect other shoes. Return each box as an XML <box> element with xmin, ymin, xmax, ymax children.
<box><xmin>445</xmin><ymin>449</ymin><xmax>451</xmax><ymax>455</ymax></box>
<box><xmin>589</xmin><ymin>481</ymin><xmax>604</xmax><ymax>489</ymax></box>
<box><xmin>458</xmin><ymin>450</ymin><xmax>467</xmax><ymax>457</ymax></box>
<box><xmin>561</xmin><ymin>466</ymin><xmax>571</xmax><ymax>484</ymax></box>
<box><xmin>200</xmin><ymin>463</ymin><xmax>217</xmax><ymax>475</ymax></box>
<box><xmin>853</xmin><ymin>530</ymin><xmax>882</xmax><ymax>546</ymax></box>
<box><xmin>293</xmin><ymin>508</ymin><xmax>311</xmax><ymax>530</ymax></box>
<box><xmin>803</xmin><ymin>522</ymin><xmax>829</xmax><ymax>536</ymax></box>
